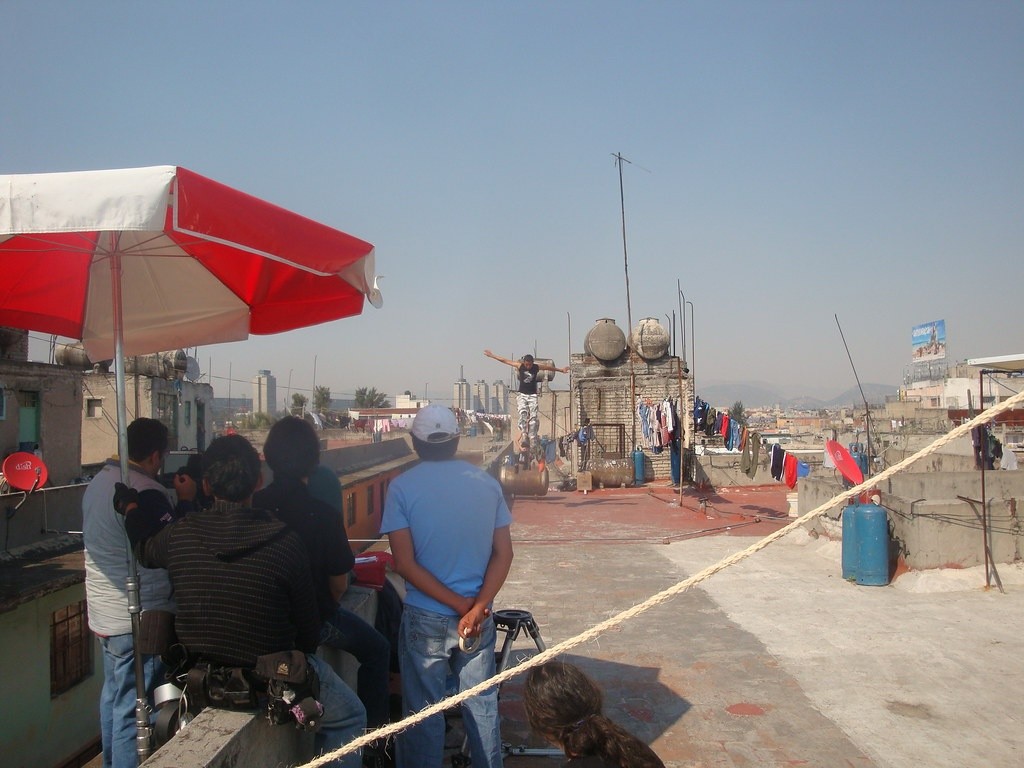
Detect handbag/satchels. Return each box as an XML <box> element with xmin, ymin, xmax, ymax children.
<box><xmin>349</xmin><ymin>551</ymin><xmax>395</xmax><ymax>591</ymax></box>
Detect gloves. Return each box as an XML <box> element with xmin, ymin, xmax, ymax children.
<box><xmin>113</xmin><ymin>481</ymin><xmax>138</xmax><ymax>515</ymax></box>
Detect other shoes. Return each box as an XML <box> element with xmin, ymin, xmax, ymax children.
<box><xmin>363</xmin><ymin>754</ymin><xmax>395</xmax><ymax>768</ymax></box>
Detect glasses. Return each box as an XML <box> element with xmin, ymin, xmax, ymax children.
<box><xmin>159</xmin><ymin>448</ymin><xmax>170</xmax><ymax>458</ymax></box>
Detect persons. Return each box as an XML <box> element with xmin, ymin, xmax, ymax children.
<box><xmin>483</xmin><ymin>350</ymin><xmax>570</xmax><ymax>447</ymax></box>
<box><xmin>380</xmin><ymin>404</ymin><xmax>514</xmax><ymax>768</ymax></box>
<box><xmin>523</xmin><ymin>662</ymin><xmax>666</xmax><ymax>768</ymax></box>
<box><xmin>81</xmin><ymin>418</ymin><xmax>197</xmax><ymax>768</ymax></box>
<box><xmin>111</xmin><ymin>434</ymin><xmax>367</xmax><ymax>768</ymax></box>
<box><xmin>251</xmin><ymin>420</ymin><xmax>390</xmax><ymax>707</ymax></box>
<box><xmin>516</xmin><ymin>433</ymin><xmax>529</xmax><ymax>470</ymax></box>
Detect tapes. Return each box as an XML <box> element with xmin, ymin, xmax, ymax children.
<box><xmin>458</xmin><ymin>627</ymin><xmax>482</xmax><ymax>654</ymax></box>
<box><xmin>152</xmin><ymin>683</ymin><xmax>186</xmax><ymax>750</ymax></box>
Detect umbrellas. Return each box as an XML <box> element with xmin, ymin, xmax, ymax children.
<box><xmin>0</xmin><ymin>165</ymin><xmax>384</xmax><ymax>763</ymax></box>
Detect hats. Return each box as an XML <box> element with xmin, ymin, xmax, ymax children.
<box><xmin>411</xmin><ymin>403</ymin><xmax>460</xmax><ymax>444</ymax></box>
